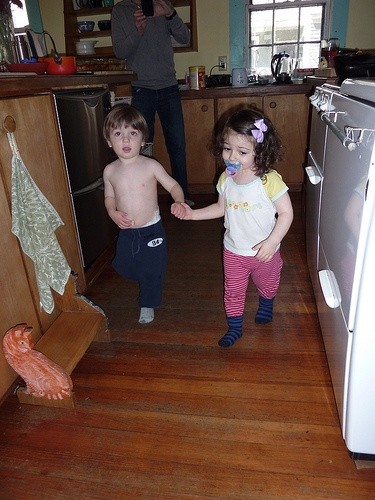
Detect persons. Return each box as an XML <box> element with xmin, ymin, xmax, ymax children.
<box><xmin>112</xmin><ymin>0</ymin><xmax>195</xmax><ymax>207</ymax></box>
<box><xmin>174</xmin><ymin>104</ymin><xmax>294</xmax><ymax>347</ymax></box>
<box><xmin>103</xmin><ymin>104</ymin><xmax>185</xmax><ymax>325</ymax></box>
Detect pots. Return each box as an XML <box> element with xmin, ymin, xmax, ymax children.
<box><xmin>39</xmin><ymin>52</ymin><xmax>77</xmax><ymax>75</ymax></box>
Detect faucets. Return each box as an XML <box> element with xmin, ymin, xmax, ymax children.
<box><xmin>40</xmin><ymin>30</ymin><xmax>61</xmax><ymax>62</ymax></box>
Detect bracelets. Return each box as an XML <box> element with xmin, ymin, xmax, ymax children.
<box><xmin>165</xmin><ymin>9</ymin><xmax>177</xmax><ymax>20</ymax></box>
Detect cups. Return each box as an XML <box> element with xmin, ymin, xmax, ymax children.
<box><xmin>75</xmin><ymin>0</ymin><xmax>114</xmax><ymax>9</ymax></box>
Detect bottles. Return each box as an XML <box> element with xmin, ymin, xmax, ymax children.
<box><xmin>320</xmin><ymin>39</ymin><xmax>328</xmax><ymax>67</ymax></box>
<box><xmin>328</xmin><ymin>37</ymin><xmax>340</xmax><ymax>69</ymax></box>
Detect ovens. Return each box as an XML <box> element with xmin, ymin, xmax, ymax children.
<box><xmin>53</xmin><ymin>87</ymin><xmax>119</xmax><ymax>272</ymax></box>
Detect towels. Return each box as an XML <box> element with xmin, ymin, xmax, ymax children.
<box><xmin>6</xmin><ymin>130</ymin><xmax>72</xmax><ymax>314</ymax></box>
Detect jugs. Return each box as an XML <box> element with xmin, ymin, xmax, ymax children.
<box><xmin>271</xmin><ymin>51</ymin><xmax>295</xmax><ymax>85</ymax></box>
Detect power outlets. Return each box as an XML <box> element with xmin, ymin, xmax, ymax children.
<box><xmin>218</xmin><ymin>56</ymin><xmax>227</xmax><ymax>70</ymax></box>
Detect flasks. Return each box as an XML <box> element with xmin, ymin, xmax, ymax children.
<box><xmin>15</xmin><ymin>34</ymin><xmax>30</xmax><ymax>62</ymax></box>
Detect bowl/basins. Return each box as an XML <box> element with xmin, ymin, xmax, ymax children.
<box><xmin>177</xmin><ymin>84</ymin><xmax>190</xmax><ymax>91</ymax></box>
<box><xmin>98</xmin><ymin>20</ymin><xmax>111</xmax><ymax>31</ymax></box>
<box><xmin>74</xmin><ymin>21</ymin><xmax>94</xmax><ymax>32</ymax></box>
<box><xmin>74</xmin><ymin>41</ymin><xmax>97</xmax><ymax>55</ymax></box>
<box><xmin>4</xmin><ymin>62</ymin><xmax>50</xmax><ymax>75</ymax></box>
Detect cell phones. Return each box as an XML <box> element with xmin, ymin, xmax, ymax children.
<box><xmin>141</xmin><ymin>0</ymin><xmax>154</xmax><ymax>17</ymax></box>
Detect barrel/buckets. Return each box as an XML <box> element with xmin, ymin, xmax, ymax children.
<box><xmin>189</xmin><ymin>66</ymin><xmax>205</xmax><ymax>90</ymax></box>
<box><xmin>232</xmin><ymin>68</ymin><xmax>248</xmax><ymax>88</ymax></box>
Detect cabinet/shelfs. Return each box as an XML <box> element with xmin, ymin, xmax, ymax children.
<box><xmin>0</xmin><ymin>90</ymin><xmax>88</xmax><ymax>409</ymax></box>
<box><xmin>157</xmin><ymin>91</ymin><xmax>312</xmax><ymax>194</ymax></box>
<box><xmin>63</xmin><ymin>0</ymin><xmax>198</xmax><ymax>59</ymax></box>
